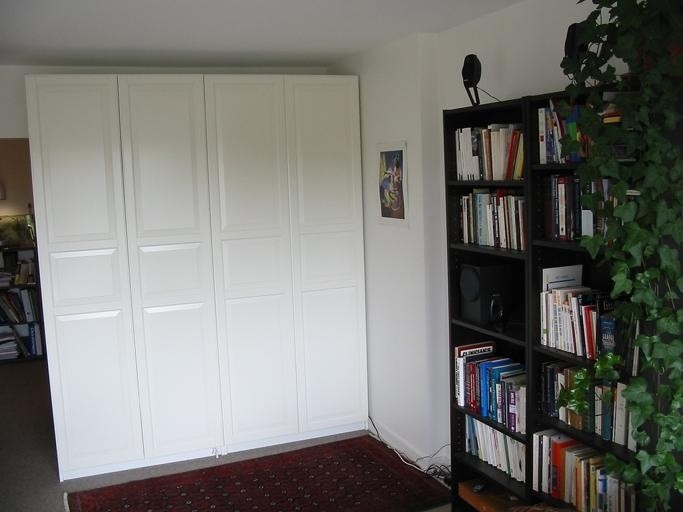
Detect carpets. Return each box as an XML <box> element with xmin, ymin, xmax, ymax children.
<box><xmin>63</xmin><ymin>435</ymin><xmax>452</xmax><ymax>512</ymax></box>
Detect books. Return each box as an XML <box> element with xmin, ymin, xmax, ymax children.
<box><xmin>453</xmin><ymin>341</ymin><xmax>656</xmax><ymax>512</ymax></box>
<box><xmin>453</xmin><ymin>123</ymin><xmax>626</xmax><ymax>252</ymax></box>
<box><xmin>540</xmin><ymin>262</ymin><xmax>647</xmax><ymax>377</ymax></box>
<box><xmin>0</xmin><ymin>247</ymin><xmax>42</xmax><ymax>361</ymax></box>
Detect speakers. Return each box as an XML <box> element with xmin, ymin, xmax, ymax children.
<box><xmin>460</xmin><ymin>262</ymin><xmax>508</xmax><ymax>325</ymax></box>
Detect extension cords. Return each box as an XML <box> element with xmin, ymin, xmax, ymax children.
<box><xmin>432</xmin><ymin>473</ymin><xmax>454</xmax><ymax>490</ymax></box>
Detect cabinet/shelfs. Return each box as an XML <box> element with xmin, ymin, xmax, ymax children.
<box><xmin>24</xmin><ymin>74</ymin><xmax>369</xmax><ymax>483</ymax></box>
<box><xmin>443</xmin><ymin>85</ymin><xmax>642</xmax><ymax>512</ymax></box>
<box><xmin>0</xmin><ymin>203</ymin><xmax>46</xmax><ymax>365</ymax></box>
